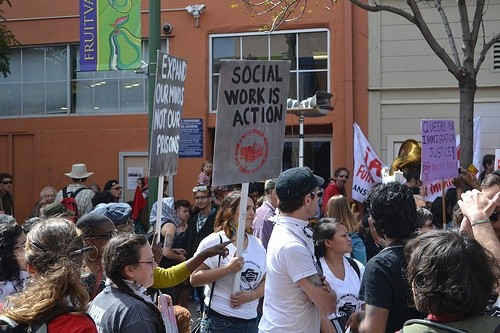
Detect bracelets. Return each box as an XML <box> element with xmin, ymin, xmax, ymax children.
<box><xmin>472</xmin><ymin>219</ymin><xmax>490</xmax><ymax>227</ymax></box>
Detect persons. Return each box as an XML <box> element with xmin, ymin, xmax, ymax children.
<box><xmin>0</xmin><ymin>154</ymin><xmax>500</xmax><ymax>333</ymax></box>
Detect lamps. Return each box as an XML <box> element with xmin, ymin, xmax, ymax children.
<box><xmin>184</xmin><ymin>3</ymin><xmax>207</xmax><ymax>18</ymax></box>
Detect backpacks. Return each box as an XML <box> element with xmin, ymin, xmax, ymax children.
<box><xmin>59</xmin><ymin>187</ymin><xmax>86</xmax><ymax>223</ymax></box>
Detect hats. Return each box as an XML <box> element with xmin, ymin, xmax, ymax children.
<box><xmin>459</xmin><ymin>172</ymin><xmax>483</xmax><ymax>192</ymax></box>
<box><xmin>94</xmin><ymin>202</ymin><xmax>132</xmax><ymax>226</ymax></box>
<box><xmin>104</xmin><ymin>180</ymin><xmax>118</xmax><ymax>190</ymax></box>
<box><xmin>64</xmin><ymin>163</ymin><xmax>95</xmax><ymax>178</ymax></box>
<box><xmin>43</xmin><ymin>203</ymin><xmax>76</xmax><ymax>218</ymax></box>
<box><xmin>265</xmin><ymin>179</ymin><xmax>276</xmax><ymax>190</ymax></box>
<box><xmin>275</xmin><ymin>167</ymin><xmax>325</xmax><ymax>198</ymax></box>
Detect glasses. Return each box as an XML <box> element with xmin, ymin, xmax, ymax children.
<box><xmin>13</xmin><ymin>240</ymin><xmax>26</xmax><ymax>251</ymax></box>
<box><xmin>420</xmin><ymin>222</ymin><xmax>433</xmax><ymax>228</ymax></box>
<box><xmin>0</xmin><ymin>181</ymin><xmax>12</xmax><ymax>184</ymax></box>
<box><xmin>489</xmin><ymin>211</ymin><xmax>500</xmax><ymax>221</ymax></box>
<box><xmin>83</xmin><ymin>230</ymin><xmax>114</xmax><ymax>239</ymax></box>
<box><xmin>193</xmin><ymin>195</ymin><xmax>210</xmax><ymax>199</ymax></box>
<box><xmin>110</xmin><ymin>187</ymin><xmax>122</xmax><ymax>190</ymax></box>
<box><xmin>364</xmin><ymin>200</ymin><xmax>386</xmax><ymax>240</ymax></box>
<box><xmin>303</xmin><ymin>187</ymin><xmax>323</xmax><ymax>197</ymax></box>
<box><xmin>127</xmin><ymin>258</ymin><xmax>154</xmax><ymax>265</ymax></box>
<box><xmin>192</xmin><ymin>185</ymin><xmax>209</xmax><ymax>193</ymax></box>
<box><xmin>338</xmin><ymin>174</ymin><xmax>348</xmax><ymax>179</ymax></box>
